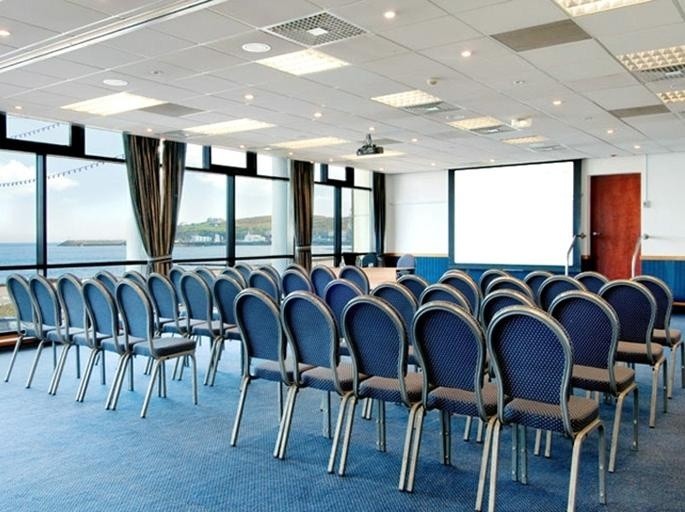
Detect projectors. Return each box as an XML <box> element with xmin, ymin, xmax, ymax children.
<box><xmin>356</xmin><ymin>144</ymin><xmax>383</xmax><ymax>155</ymax></box>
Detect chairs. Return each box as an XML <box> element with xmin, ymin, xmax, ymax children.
<box><xmin>123</xmin><ymin>270</ymin><xmax>186</xmax><ymax>375</ymax></box>
<box><xmin>169</xmin><ymin>274</ymin><xmax>245</xmax><ymax>321</ymax></box>
<box><xmin>51</xmin><ymin>273</ymin><xmax>105</xmax><ymax>403</ymax></box>
<box><xmin>5</xmin><ymin>274</ymin><xmax>78</xmax><ymax>388</ymax></box>
<box><xmin>362</xmin><ymin>254</ymin><xmax>377</xmax><ymax>267</ymax></box>
<box><xmin>209</xmin><ymin>277</ymin><xmax>245</xmax><ymax>387</ymax></box>
<box><xmin>634</xmin><ymin>276</ymin><xmax>684</xmax><ymax>398</ymax></box>
<box><xmin>397</xmin><ymin>254</ymin><xmax>415</xmax><ymax>274</ymax></box>
<box><xmin>365</xmin><ymin>281</ymin><xmax>420</xmax><ymax>431</ymax></box>
<box><xmin>337</xmin><ymin>295</ymin><xmax>445</xmax><ymax>492</ymax></box>
<box><xmin>320</xmin><ymin>276</ymin><xmax>371</xmax><ymax>420</ymax></box>
<box><xmin>413</xmin><ymin>268</ymin><xmax>606</xmax><ymax>289</ymax></box>
<box><xmin>413</xmin><ymin>283</ymin><xmax>475</xmax><ymax>443</ymax></box>
<box><xmin>178</xmin><ymin>272</ymin><xmax>236</xmax><ymax>385</ymax></box>
<box><xmin>327</xmin><ymin>268</ymin><xmax>412</xmax><ymax>289</ymax></box>
<box><xmin>597</xmin><ymin>279</ymin><xmax>668</xmax><ymax>428</ymax></box>
<box><xmin>170</xmin><ymin>262</ymin><xmax>247</xmax><ymax>274</ymax></box>
<box><xmin>94</xmin><ymin>271</ymin><xmax>118</xmax><ymax>365</ymax></box>
<box><xmin>406</xmin><ymin>300</ymin><xmax>517</xmax><ymax>512</ymax></box>
<box><xmin>25</xmin><ymin>274</ymin><xmax>80</xmax><ymax>395</ymax></box>
<box><xmin>475</xmin><ymin>290</ymin><xmax>544</xmax><ymax>456</ymax></box>
<box><xmin>487</xmin><ymin>304</ymin><xmax>606</xmax><ymax>512</ymax></box>
<box><xmin>147</xmin><ymin>273</ymin><xmax>213</xmax><ymax>381</ymax></box>
<box><xmin>278</xmin><ymin>290</ymin><xmax>380</xmax><ymax>474</ymax></box>
<box><xmin>79</xmin><ymin>279</ymin><xmax>161</xmax><ymax>410</ymax></box>
<box><xmin>247</xmin><ymin>263</ymin><xmax>326</xmax><ymax>298</ymax></box>
<box><xmin>110</xmin><ymin>280</ymin><xmax>197</xmax><ymax>418</ymax></box>
<box><xmin>547</xmin><ymin>290</ymin><xmax>639</xmax><ymax>472</ymax></box>
<box><xmin>229</xmin><ymin>287</ymin><xmax>325</xmax><ymax>458</ymax></box>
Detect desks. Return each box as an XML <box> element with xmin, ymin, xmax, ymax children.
<box><xmin>325</xmin><ymin>267</ymin><xmax>413</xmax><ymax>290</ymax></box>
